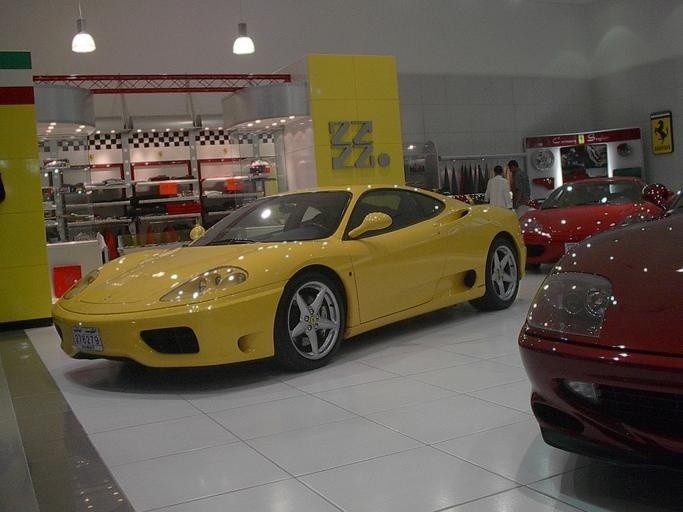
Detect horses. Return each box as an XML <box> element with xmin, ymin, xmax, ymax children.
<box><xmin>655</xmin><ymin>120</ymin><xmax>669</xmax><ymax>144</ymax></box>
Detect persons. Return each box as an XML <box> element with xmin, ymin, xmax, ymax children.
<box><xmin>507</xmin><ymin>159</ymin><xmax>531</xmax><ymax>220</ymax></box>
<box><xmin>482</xmin><ymin>165</ymin><xmax>513</xmax><ymax>210</ymax></box>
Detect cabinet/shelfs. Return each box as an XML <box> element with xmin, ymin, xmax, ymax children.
<box><xmin>65</xmin><ymin>175</ymin><xmax>265</xmax><ymax>226</ymax></box>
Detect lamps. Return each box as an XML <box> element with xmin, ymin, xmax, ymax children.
<box><xmin>70</xmin><ymin>0</ymin><xmax>97</xmax><ymax>54</ymax></box>
<box><xmin>232</xmin><ymin>0</ymin><xmax>257</xmax><ymax>56</ymax></box>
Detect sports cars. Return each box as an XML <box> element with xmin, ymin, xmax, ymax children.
<box><xmin>513</xmin><ymin>182</ymin><xmax>683</xmax><ymax>477</ymax></box>
<box><xmin>517</xmin><ymin>175</ymin><xmax>673</xmax><ymax>270</ymax></box>
<box><xmin>49</xmin><ymin>185</ymin><xmax>528</xmax><ymax>375</ymax></box>
